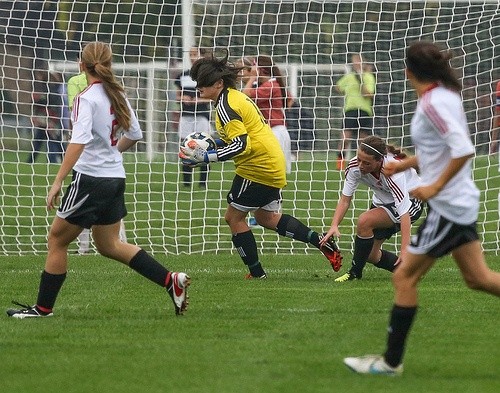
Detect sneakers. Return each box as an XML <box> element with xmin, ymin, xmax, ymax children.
<box><xmin>319</xmin><ymin>232</ymin><xmax>343</xmax><ymax>272</ymax></box>
<box><xmin>334</xmin><ymin>272</ymin><xmax>362</xmax><ymax>282</ymax></box>
<box><xmin>166</xmin><ymin>272</ymin><xmax>191</xmax><ymax>317</ymax></box>
<box><xmin>343</xmin><ymin>355</ymin><xmax>403</xmax><ymax>376</ymax></box>
<box><xmin>244</xmin><ymin>273</ymin><xmax>267</xmax><ymax>280</ymax></box>
<box><xmin>6</xmin><ymin>303</ymin><xmax>54</xmax><ymax>319</ymax></box>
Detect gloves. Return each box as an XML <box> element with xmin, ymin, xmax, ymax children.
<box><xmin>178</xmin><ymin>139</ymin><xmax>222</xmax><ymax>168</ymax></box>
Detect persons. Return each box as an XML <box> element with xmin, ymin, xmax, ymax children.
<box><xmin>342</xmin><ymin>42</ymin><xmax>500</xmax><ymax>376</ymax></box>
<box><xmin>7</xmin><ymin>41</ymin><xmax>192</xmax><ymax>321</ymax></box>
<box><xmin>319</xmin><ymin>135</ymin><xmax>426</xmax><ymax>282</ymax></box>
<box><xmin>0</xmin><ymin>52</ymin><xmax>500</xmax><ymax>230</ymax></box>
<box><xmin>177</xmin><ymin>51</ymin><xmax>343</xmax><ymax>282</ymax></box>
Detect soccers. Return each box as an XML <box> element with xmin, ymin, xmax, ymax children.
<box><xmin>181</xmin><ymin>131</ymin><xmax>217</xmax><ymax>151</ymax></box>
